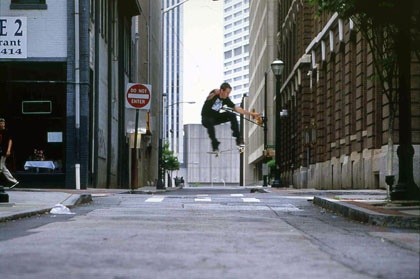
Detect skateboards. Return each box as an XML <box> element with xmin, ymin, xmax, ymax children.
<box><xmin>207</xmin><ymin>146</ymin><xmax>245</xmax><ymax>156</ymax></box>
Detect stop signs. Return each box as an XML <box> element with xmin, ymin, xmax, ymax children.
<box><xmin>125</xmin><ymin>83</ymin><xmax>152</xmax><ymax>109</ymax></box>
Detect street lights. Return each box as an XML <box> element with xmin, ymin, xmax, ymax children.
<box><xmin>270</xmin><ymin>58</ymin><xmax>286</xmax><ymax>187</ymax></box>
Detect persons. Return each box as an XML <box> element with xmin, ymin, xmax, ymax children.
<box><xmin>201</xmin><ymin>83</ymin><xmax>261</xmax><ymax>152</ymax></box>
<box><xmin>0</xmin><ymin>119</ymin><xmax>19</xmax><ymax>189</ymax></box>
